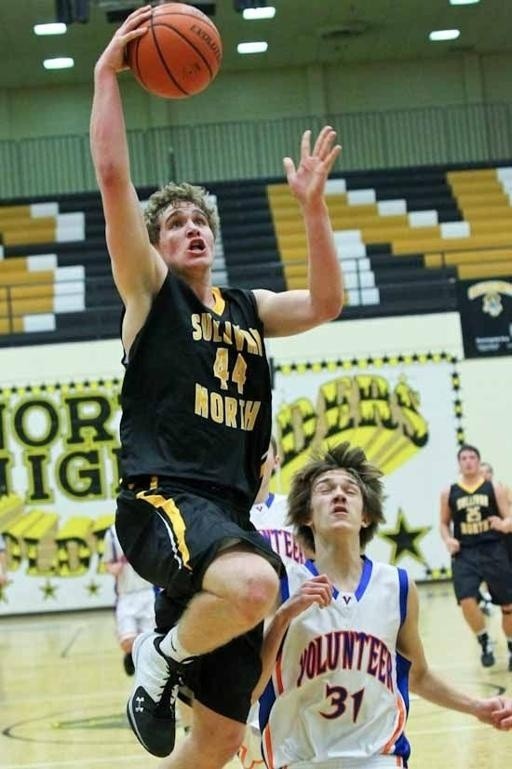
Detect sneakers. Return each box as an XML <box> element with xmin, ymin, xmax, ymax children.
<box><xmin>478</xmin><ymin>634</ymin><xmax>494</xmax><ymax>667</ymax></box>
<box><xmin>127</xmin><ymin>632</ymin><xmax>199</xmax><ymax>757</ymax></box>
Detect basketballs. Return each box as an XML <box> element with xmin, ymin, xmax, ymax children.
<box><xmin>127</xmin><ymin>3</ymin><xmax>223</xmax><ymax>99</ymax></box>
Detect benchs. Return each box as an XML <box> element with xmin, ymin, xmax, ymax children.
<box><xmin>0</xmin><ymin>150</ymin><xmax>512</xmax><ymax>355</ymax></box>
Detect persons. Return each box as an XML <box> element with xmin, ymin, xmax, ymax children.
<box><xmin>440</xmin><ymin>445</ymin><xmax>512</xmax><ymax>673</ymax></box>
<box><xmin>242</xmin><ymin>436</ymin><xmax>310</xmax><ymax>769</ymax></box>
<box><xmin>0</xmin><ymin>534</ymin><xmax>9</xmax><ymax>591</ymax></box>
<box><xmin>477</xmin><ymin>463</ymin><xmax>512</xmax><ymax>616</ymax></box>
<box><xmin>251</xmin><ymin>442</ymin><xmax>512</xmax><ymax>769</ymax></box>
<box><xmin>90</xmin><ymin>2</ymin><xmax>345</xmax><ymax>769</ymax></box>
<box><xmin>100</xmin><ymin>523</ymin><xmax>157</xmax><ymax>677</ymax></box>
